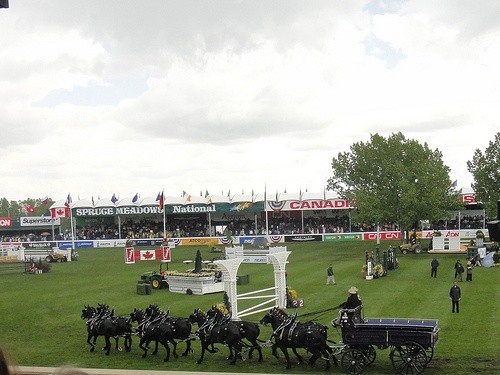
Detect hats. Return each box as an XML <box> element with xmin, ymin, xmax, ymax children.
<box><xmin>467</xmin><ymin>261</ymin><xmax>471</xmax><ymax>265</ymax></box>
<box><xmin>348</xmin><ymin>286</ymin><xmax>359</xmax><ymax>294</ymax></box>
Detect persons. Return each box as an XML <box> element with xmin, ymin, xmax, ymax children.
<box><xmin>223</xmin><ymin>214</ymin><xmax>399</xmax><ymax>236</ymax></box>
<box><xmin>454</xmin><ymin>257</ymin><xmax>475</xmax><ymax>282</ymax></box>
<box><xmin>449</xmin><ymin>281</ymin><xmax>461</xmax><ymax>313</ymax></box>
<box><xmin>326</xmin><ymin>264</ymin><xmax>336</xmax><ymax>285</ymax></box>
<box><xmin>489</xmin><ymin>243</ymin><xmax>499</xmax><ymax>253</ymax></box>
<box><xmin>431</xmin><ymin>257</ymin><xmax>439</xmax><ymax>278</ymax></box>
<box><xmin>332</xmin><ymin>287</ymin><xmax>361</xmax><ymax>326</ymax></box>
<box><xmin>0</xmin><ymin>213</ymin><xmax>209</xmax><ymax>242</ymax></box>
<box><xmin>410</xmin><ymin>214</ymin><xmax>490</xmax><ymax>230</ymax></box>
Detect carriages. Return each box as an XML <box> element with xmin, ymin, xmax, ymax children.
<box><xmin>80</xmin><ymin>302</ymin><xmax>442</xmax><ymax>375</ymax></box>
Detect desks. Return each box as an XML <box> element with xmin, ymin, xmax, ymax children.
<box><xmin>484</xmin><ymin>243</ymin><xmax>492</xmax><ymax>245</ymax></box>
<box><xmin>183</xmin><ymin>260</ymin><xmax>217</xmax><ymax>269</ymax></box>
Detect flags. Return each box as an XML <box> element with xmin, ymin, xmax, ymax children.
<box><xmin>25</xmin><ymin>205</ymin><xmax>36</xmax><ymax>213</ymax></box>
<box><xmin>41</xmin><ymin>198</ymin><xmax>49</xmax><ymax>206</ymax></box>
<box><xmin>65</xmin><ymin>188</ymin><xmax>279</xmax><ymax>208</ymax></box>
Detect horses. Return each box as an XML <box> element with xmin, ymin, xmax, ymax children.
<box><xmin>125</xmin><ymin>307</ymin><xmax>180</xmax><ymax>362</ymax></box>
<box><xmin>144</xmin><ymin>304</ymin><xmax>192</xmax><ymax>357</ymax></box>
<box><xmin>204</xmin><ymin>304</ymin><xmax>267</xmax><ymax>364</ymax></box>
<box><xmin>259</xmin><ymin>306</ymin><xmax>340</xmax><ymax>373</ymax></box>
<box><xmin>80</xmin><ymin>303</ymin><xmax>119</xmax><ymax>356</ymax></box>
<box><xmin>94</xmin><ymin>301</ymin><xmax>133</xmax><ymax>353</ymax></box>
<box><xmin>185</xmin><ymin>307</ymin><xmax>245</xmax><ymax>366</ymax></box>
